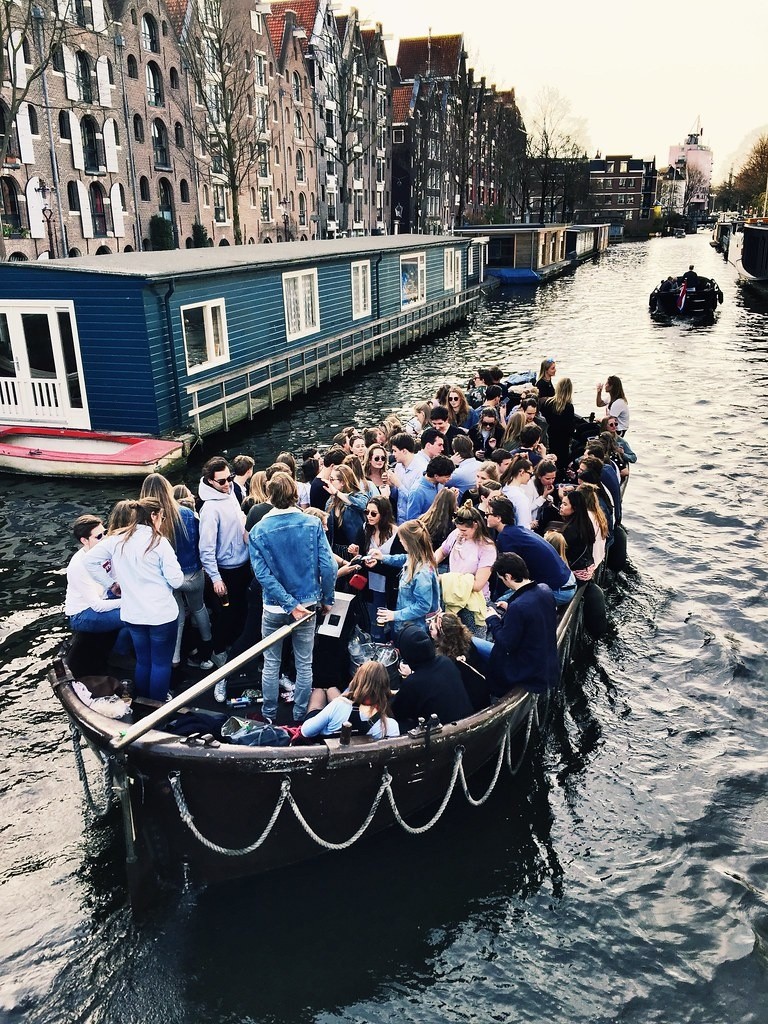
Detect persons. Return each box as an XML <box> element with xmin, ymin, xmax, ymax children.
<box><xmin>660</xmin><ymin>276</ymin><xmax>674</xmax><ymax>291</ymax></box>
<box><xmin>301</xmin><ymin>661</ymin><xmax>400</xmax><ymax>740</ymax></box>
<box><xmin>107</xmin><ymin>359</ymin><xmax>636</xmax><ymax>712</ymax></box>
<box><xmin>112</xmin><ymin>497</ymin><xmax>184</xmax><ymax>731</ymax></box>
<box><xmin>65</xmin><ymin>514</ymin><xmax>137</xmax><ymax>671</ymax></box>
<box><xmin>247</xmin><ymin>471</ymin><xmax>335</xmax><ymax>721</ymax></box>
<box><xmin>681</xmin><ymin>265</ymin><xmax>697</xmax><ymax>285</ymax></box>
<box><xmin>677</xmin><ymin>279</ymin><xmax>687</xmax><ymax>313</ymax></box>
<box><xmin>389</xmin><ymin>624</ymin><xmax>474</xmax><ymax>730</ymax></box>
<box><xmin>575</xmin><ymin>376</ymin><xmax>629</xmax><ymax>442</ymax></box>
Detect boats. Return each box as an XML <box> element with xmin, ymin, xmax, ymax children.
<box><xmin>447</xmin><ymin>224</ymin><xmax>611</xmax><ymax>283</ymax></box>
<box><xmin>0</xmin><ymin>230</ymin><xmax>503</xmax><ymax>481</ymax></box>
<box><xmin>652</xmin><ymin>275</ymin><xmax>725</xmax><ymax>319</ymax></box>
<box><xmin>38</xmin><ymin>375</ymin><xmax>631</xmax><ymax>911</ymax></box>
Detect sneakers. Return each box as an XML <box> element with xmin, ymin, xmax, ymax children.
<box><xmin>279</xmin><ymin>673</ymin><xmax>296</xmax><ymax>690</ymax></box>
<box><xmin>213</xmin><ymin>679</ymin><xmax>228</xmax><ymax>702</ymax></box>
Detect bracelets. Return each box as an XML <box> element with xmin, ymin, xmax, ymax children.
<box><xmin>340</xmin><ymin>558</ymin><xmax>344</xmax><ymax>566</ymax></box>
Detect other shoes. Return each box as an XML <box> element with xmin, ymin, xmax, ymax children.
<box><xmin>248</xmin><ymin>710</ymin><xmax>273</xmax><ymax>724</ymax></box>
<box><xmin>211</xmin><ymin>649</ymin><xmax>228</xmax><ymax>667</ymax></box>
<box><xmin>287</xmin><ymin>719</ymin><xmax>302</xmax><ymax>725</ymax></box>
<box><xmin>107</xmin><ymin>651</ymin><xmax>137</xmax><ymax>670</ymax></box>
<box><xmin>187</xmin><ymin>655</ymin><xmax>213</xmax><ymax>669</ymax></box>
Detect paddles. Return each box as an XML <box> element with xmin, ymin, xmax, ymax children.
<box><xmin>109</xmin><ymin>609</ymin><xmax>318</xmax><ymax>751</ymax></box>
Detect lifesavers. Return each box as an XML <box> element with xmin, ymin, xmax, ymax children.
<box><xmin>724</xmin><ymin>251</ymin><xmax>728</xmax><ymax>259</ymax></box>
<box><xmin>716</xmin><ymin>244</ymin><xmax>722</xmax><ymax>253</ymax></box>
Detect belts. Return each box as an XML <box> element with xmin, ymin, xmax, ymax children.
<box><xmin>555</xmin><ymin>583</ymin><xmax>576</xmax><ymax>590</ymax></box>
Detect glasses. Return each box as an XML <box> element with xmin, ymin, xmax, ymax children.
<box><xmin>450</xmin><ymin>512</ymin><xmax>471</xmax><ymax>524</ymax></box>
<box><xmin>330</xmin><ymin>477</ymin><xmax>340</xmax><ymax>482</ymax></box>
<box><xmin>448</xmin><ymin>397</ymin><xmax>460</xmax><ymax>401</ymax></box>
<box><xmin>474</xmin><ymin>377</ymin><xmax>481</xmax><ymax>381</ymax></box>
<box><xmin>607</xmin><ymin>422</ymin><xmax>617</xmax><ymax>427</ymax></box>
<box><xmin>484</xmin><ymin>513</ymin><xmax>493</xmax><ymax>518</ymax></box>
<box><xmin>519</xmin><ymin>470</ymin><xmax>534</xmax><ymax>478</ymax></box>
<box><xmin>372</xmin><ymin>456</ymin><xmax>386</xmax><ymax>461</ymax></box>
<box><xmin>482</xmin><ymin>422</ymin><xmax>494</xmax><ymax>427</ymax></box>
<box><xmin>88</xmin><ymin>529</ymin><xmax>108</xmax><ymax>541</ymax></box>
<box><xmin>210</xmin><ymin>475</ymin><xmax>233</xmax><ymax>485</ymax></box>
<box><xmin>158</xmin><ymin>513</ymin><xmax>166</xmax><ymax>522</ymax></box>
<box><xmin>363</xmin><ymin>509</ymin><xmax>378</xmax><ymax>517</ymax></box>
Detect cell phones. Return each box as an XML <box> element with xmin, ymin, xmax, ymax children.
<box><xmin>486</xmin><ymin>599</ymin><xmax>507</xmax><ymax>616</ymax></box>
